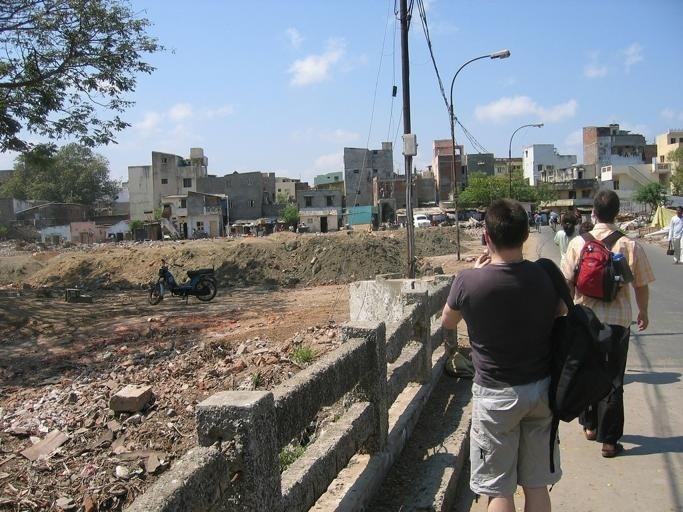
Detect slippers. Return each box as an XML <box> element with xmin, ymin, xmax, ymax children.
<box><xmin>584</xmin><ymin>426</ymin><xmax>597</xmax><ymax>440</ymax></box>
<box><xmin>601</xmin><ymin>443</ymin><xmax>623</xmax><ymax>457</ymax></box>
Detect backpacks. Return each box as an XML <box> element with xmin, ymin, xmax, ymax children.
<box><xmin>575</xmin><ymin>230</ymin><xmax>626</xmax><ymax>302</ymax></box>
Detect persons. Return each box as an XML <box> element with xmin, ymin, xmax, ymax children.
<box><xmin>666</xmin><ymin>205</ymin><xmax>683</xmax><ymax>265</ymax></box>
<box><xmin>559</xmin><ymin>185</ymin><xmax>659</xmax><ymax>458</ymax></box>
<box><xmin>526</xmin><ymin>203</ymin><xmax>595</xmax><ymax>261</ymax></box>
<box><xmin>441</xmin><ymin>197</ymin><xmax>572</xmax><ymax>512</ymax></box>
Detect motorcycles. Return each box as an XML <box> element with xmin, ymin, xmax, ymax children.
<box><xmin>147</xmin><ymin>258</ymin><xmax>218</xmax><ymax>305</ymax></box>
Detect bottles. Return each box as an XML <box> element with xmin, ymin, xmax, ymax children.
<box><xmin>612</xmin><ymin>252</ymin><xmax>635</xmax><ymax>283</ymax></box>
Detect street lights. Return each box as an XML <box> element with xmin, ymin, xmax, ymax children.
<box><xmin>510</xmin><ymin>122</ymin><xmax>545</xmax><ymax>200</ymax></box>
<box><xmin>449</xmin><ymin>49</ymin><xmax>512</xmax><ymax>261</ymax></box>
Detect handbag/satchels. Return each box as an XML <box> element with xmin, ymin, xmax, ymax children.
<box><xmin>667</xmin><ymin>250</ymin><xmax>674</xmax><ymax>254</ymax></box>
<box><xmin>549</xmin><ymin>303</ymin><xmax>623</xmax><ymax>422</ymax></box>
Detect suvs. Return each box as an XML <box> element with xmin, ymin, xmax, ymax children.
<box><xmin>427</xmin><ymin>213</ymin><xmax>455</xmax><ymax>227</ymax></box>
<box><xmin>413</xmin><ymin>213</ymin><xmax>432</xmax><ymax>229</ymax></box>
